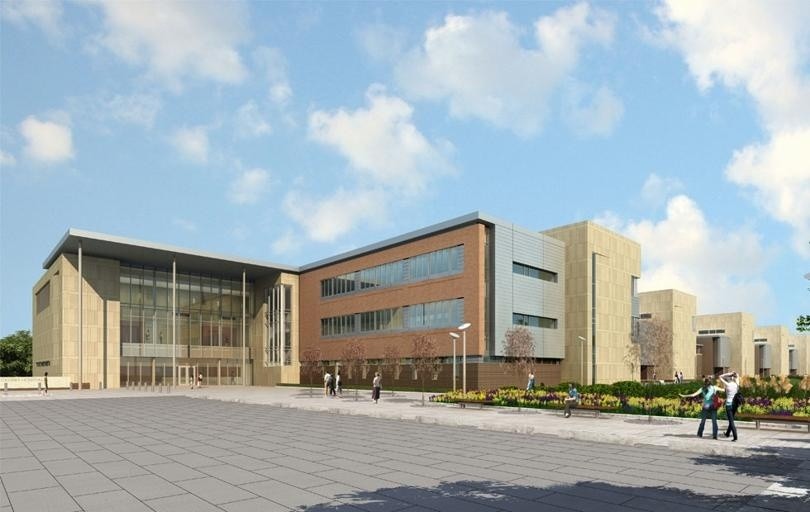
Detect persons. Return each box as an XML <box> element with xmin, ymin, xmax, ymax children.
<box><xmin>674</xmin><ymin>371</ymin><xmax>678</xmax><ymax>383</ymax></box>
<box><xmin>336</xmin><ymin>373</ymin><xmax>342</xmax><ymax>393</ymax></box>
<box><xmin>372</xmin><ymin>371</ymin><xmax>382</xmax><ymax>404</ymax></box>
<box><xmin>197</xmin><ymin>372</ymin><xmax>203</xmax><ymax>386</ymax></box>
<box><xmin>680</xmin><ymin>376</ymin><xmax>725</xmax><ymax>438</ymax></box>
<box><xmin>324</xmin><ymin>370</ymin><xmax>331</xmax><ymax>394</ymax></box>
<box><xmin>719</xmin><ymin>371</ymin><xmax>740</xmax><ymax>440</ymax></box>
<box><xmin>680</xmin><ymin>371</ymin><xmax>683</xmax><ymax>380</ymax></box>
<box><xmin>526</xmin><ymin>372</ymin><xmax>535</xmax><ymax>389</ymax></box>
<box><xmin>562</xmin><ymin>381</ymin><xmax>578</xmax><ymax>419</ymax></box>
<box><xmin>327</xmin><ymin>374</ymin><xmax>336</xmax><ymax>396</ymax></box>
